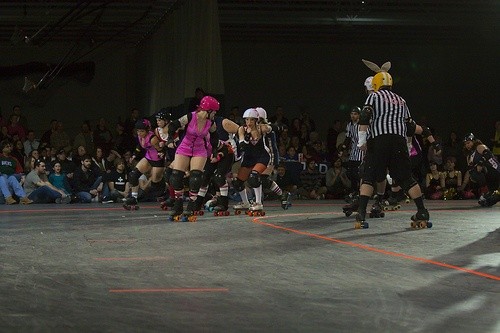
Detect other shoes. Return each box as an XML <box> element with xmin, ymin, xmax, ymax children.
<box><xmin>318</xmin><ymin>194</ymin><xmax>325</xmax><ymax>200</ymax></box>
<box><xmin>19</xmin><ymin>197</ymin><xmax>33</xmax><ymax>204</ymax></box>
<box><xmin>55</xmin><ymin>197</ymin><xmax>71</xmax><ymax>203</ymax></box>
<box><xmin>293</xmin><ymin>194</ymin><xmax>300</xmax><ymax>199</ymax></box>
<box><xmin>102</xmin><ymin>197</ymin><xmax>114</xmax><ymax>203</ymax></box>
<box><xmin>5</xmin><ymin>197</ymin><xmax>16</xmax><ymax>204</ymax></box>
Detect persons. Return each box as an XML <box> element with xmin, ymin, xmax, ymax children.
<box><xmin>0</xmin><ymin>76</ymin><xmax>500</xmax><ymax>221</ymax></box>
<box><xmin>353</xmin><ymin>71</ymin><xmax>433</xmax><ymax>228</ymax></box>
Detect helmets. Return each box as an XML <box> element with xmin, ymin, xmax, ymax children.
<box><xmin>155</xmin><ymin>112</ymin><xmax>171</xmax><ymax>121</ymax></box>
<box><xmin>200</xmin><ymin>96</ymin><xmax>219</xmax><ymax>111</ymax></box>
<box><xmin>364</xmin><ymin>72</ymin><xmax>392</xmax><ymax>92</ymax></box>
<box><xmin>243</xmin><ymin>107</ymin><xmax>266</xmax><ymax>119</ymax></box>
<box><xmin>351</xmin><ymin>106</ymin><xmax>362</xmax><ymax>114</ymax></box>
<box><xmin>464</xmin><ymin>133</ymin><xmax>474</xmax><ymax>141</ymax></box>
<box><xmin>136</xmin><ymin>120</ymin><xmax>151</xmax><ymax>130</ymax></box>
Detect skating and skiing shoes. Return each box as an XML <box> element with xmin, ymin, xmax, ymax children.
<box><xmin>123</xmin><ymin>197</ymin><xmax>139</xmax><ymax>211</ymax></box>
<box><xmin>369</xmin><ymin>202</ymin><xmax>385</xmax><ymax>218</ymax></box>
<box><xmin>410</xmin><ymin>209</ymin><xmax>432</xmax><ymax>229</ymax></box>
<box><xmin>233</xmin><ymin>202</ymin><xmax>250</xmax><ymax>215</ymax></box>
<box><xmin>353</xmin><ymin>214</ymin><xmax>369</xmax><ymax>230</ymax></box>
<box><xmin>248</xmin><ymin>204</ymin><xmax>265</xmax><ymax>216</ymax></box>
<box><xmin>383</xmin><ymin>197</ymin><xmax>401</xmax><ymax>211</ymax></box>
<box><xmin>342</xmin><ymin>200</ymin><xmax>360</xmax><ymax>217</ymax></box>
<box><xmin>280</xmin><ymin>192</ymin><xmax>291</xmax><ymax>210</ymax></box>
<box><xmin>160</xmin><ymin>199</ymin><xmax>229</xmax><ymax>222</ymax></box>
